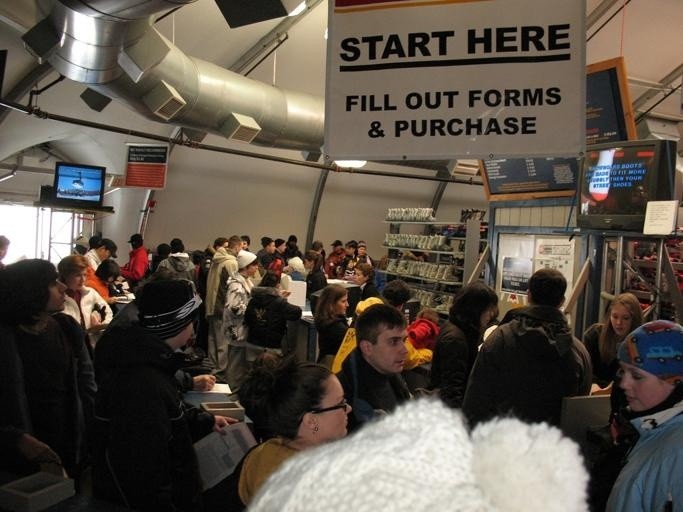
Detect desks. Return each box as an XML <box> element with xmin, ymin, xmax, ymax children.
<box><xmin>326</xmin><ymin>279</ymin><xmax>360</xmax><ymax>316</ymax></box>
<box><xmin>297</xmin><ymin>306</ymin><xmax>320</xmax><ymax>363</ymax></box>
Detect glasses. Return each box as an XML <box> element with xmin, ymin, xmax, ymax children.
<box><xmin>311</xmin><ymin>399</ymin><xmax>348</xmax><ymax>413</ymax></box>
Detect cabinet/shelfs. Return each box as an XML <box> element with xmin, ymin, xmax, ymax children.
<box><xmin>623</xmin><ymin>237</ymin><xmax>683</xmax><ymax>324</ymax></box>
<box><xmin>383</xmin><ymin>208</ymin><xmax>489</xmax><ymax>316</ymax></box>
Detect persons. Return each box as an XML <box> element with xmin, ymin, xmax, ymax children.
<box><xmin>120</xmin><ymin>234</ymin><xmax>149</xmax><ymax>289</ymax></box>
<box><xmin>283</xmin><ymin>244</ymin><xmax>305</xmax><ymax>275</ymax></box>
<box><xmin>325</xmin><ymin>240</ymin><xmax>348</xmax><ymax>280</ymax></box>
<box><xmin>287</xmin><ymin>234</ymin><xmax>297</xmax><ymax>245</ymax></box>
<box><xmin>354</xmin><ymin>264</ymin><xmax>378</xmax><ymax>300</ymax></box>
<box><xmin>331</xmin><ymin>279</ymin><xmax>433</xmax><ymax>377</ymax></box>
<box><xmin>154</xmin><ymin>237</ymin><xmax>194</xmax><ymax>287</ymax></box>
<box><xmin>238</xmin><ymin>362</ymin><xmax>352</xmax><ymax>512</ymax></box>
<box><xmin>605</xmin><ymin>320</ymin><xmax>683</xmax><ymax>512</ymax></box>
<box><xmin>461</xmin><ymin>267</ymin><xmax>591</xmax><ymax>429</ymax></box>
<box><xmin>429</xmin><ymin>281</ymin><xmax>495</xmax><ymax>408</ymax></box>
<box><xmin>58</xmin><ymin>255</ymin><xmax>114</xmax><ymax>360</ymax></box>
<box><xmin>407</xmin><ymin>308</ymin><xmax>441</xmax><ymax>351</ymax></box>
<box><xmin>336</xmin><ymin>303</ymin><xmax>416</xmax><ymax>434</ymax></box>
<box><xmin>304</xmin><ymin>251</ymin><xmax>328</xmax><ymax>317</ymax></box>
<box><xmin>0</xmin><ymin>259</ymin><xmax>93</xmax><ymax>512</ymax></box>
<box><xmin>240</xmin><ymin>235</ymin><xmax>250</xmax><ymax>252</ymax></box>
<box><xmin>151</xmin><ymin>243</ymin><xmax>171</xmax><ymax>275</ymax></box>
<box><xmin>190</xmin><ymin>249</ymin><xmax>204</xmax><ymax>283</ymax></box>
<box><xmin>199</xmin><ymin>237</ymin><xmax>229</xmax><ymax>357</ymax></box>
<box><xmin>272</xmin><ymin>239</ymin><xmax>287</xmax><ymax>261</ymax></box>
<box><xmin>94</xmin><ymin>278</ymin><xmax>241</xmax><ymax>512</ymax></box>
<box><xmin>223</xmin><ymin>250</ymin><xmax>258</xmax><ymax>401</ymax></box>
<box><xmin>334</xmin><ymin>241</ymin><xmax>359</xmax><ymax>272</ymax></box>
<box><xmin>0</xmin><ymin>235</ymin><xmax>10</xmax><ymax>271</ymax></box>
<box><xmin>583</xmin><ymin>293</ymin><xmax>644</xmax><ymax>395</ymax></box>
<box><xmin>315</xmin><ymin>285</ymin><xmax>349</xmax><ymax>351</ymax></box>
<box><xmin>341</xmin><ymin>258</ymin><xmax>355</xmax><ymax>281</ymax></box>
<box><xmin>243</xmin><ymin>271</ymin><xmax>302</xmax><ymax>372</ymax></box>
<box><xmin>85</xmin><ymin>260</ymin><xmax>119</xmax><ymax>306</ymax></box>
<box><xmin>261</xmin><ymin>238</ymin><xmax>277</xmax><ymax>269</ymax></box>
<box><xmin>92</xmin><ymin>287</ymin><xmax>216</xmax><ymax>394</ymax></box>
<box><xmin>355</xmin><ymin>241</ymin><xmax>374</xmax><ymax>267</ymax></box>
<box><xmin>205</xmin><ymin>235</ymin><xmax>240</xmax><ymax>366</ymax></box>
<box><xmin>83</xmin><ymin>239</ymin><xmax>117</xmax><ymax>270</ymax></box>
<box><xmin>307</xmin><ymin>241</ymin><xmax>323</xmax><ymax>273</ymax></box>
<box><xmin>73</xmin><ymin>236</ymin><xmax>89</xmax><ymax>256</ymax></box>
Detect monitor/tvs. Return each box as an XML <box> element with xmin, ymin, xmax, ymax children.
<box><xmin>578</xmin><ymin>140</ymin><xmax>676</xmax><ymax>232</ymax></box>
<box><xmin>54</xmin><ymin>162</ymin><xmax>107</xmax><ymax>204</ymax></box>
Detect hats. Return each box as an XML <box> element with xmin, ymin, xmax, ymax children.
<box><xmin>331</xmin><ymin>240</ymin><xmax>342</xmax><ymax>246</ymax></box>
<box><xmin>615</xmin><ymin>319</ymin><xmax>683</xmax><ymax>384</ymax></box>
<box><xmin>237</xmin><ymin>250</ymin><xmax>258</xmax><ymax>268</ymax></box>
<box><xmin>127</xmin><ymin>234</ymin><xmax>143</xmax><ymax>248</ymax></box>
<box><xmin>261</xmin><ymin>236</ymin><xmax>275</xmax><ymax>247</ymax></box>
<box><xmin>358</xmin><ymin>241</ymin><xmax>367</xmax><ymax>247</ymax></box>
<box><xmin>135</xmin><ymin>277</ymin><xmax>204</xmax><ymax>339</ymax></box>
<box><xmin>288</xmin><ymin>235</ymin><xmax>297</xmax><ymax>243</ymax></box>
<box><xmin>275</xmin><ymin>239</ymin><xmax>285</xmax><ymax>247</ymax></box>
<box><xmin>98</xmin><ymin>238</ymin><xmax>118</xmax><ymax>258</ymax></box>
